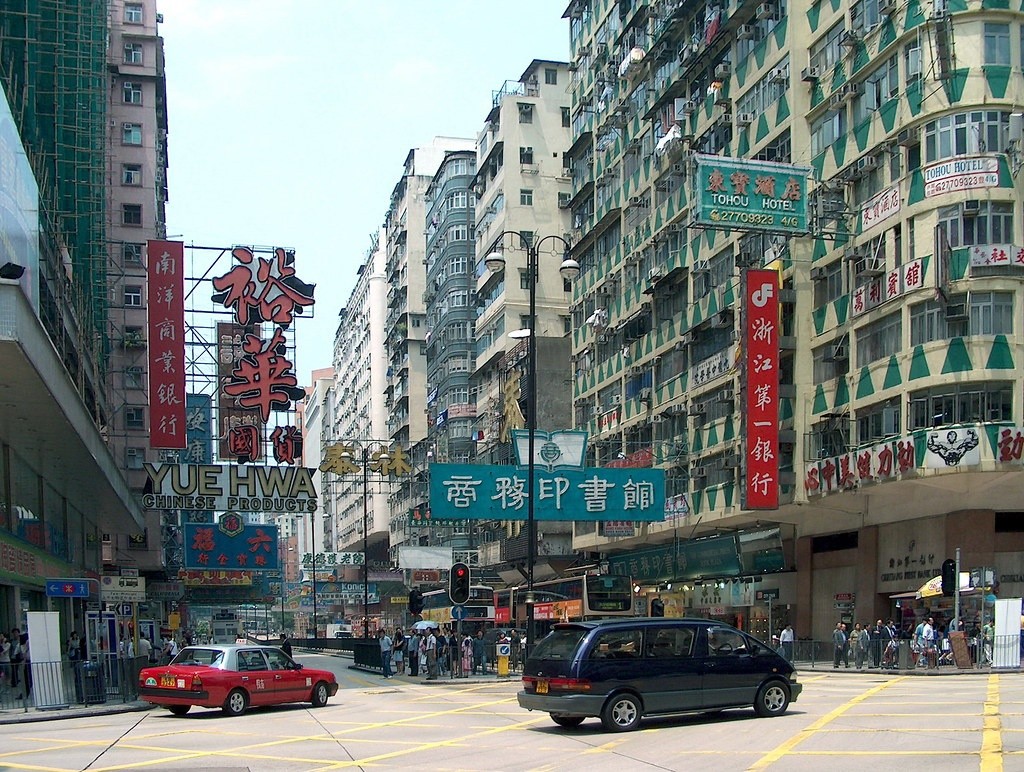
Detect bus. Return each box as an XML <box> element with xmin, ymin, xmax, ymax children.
<box><xmin>489</xmin><ymin>572</ymin><xmax>635</xmax><ymax>647</ymax></box>
<box><xmin>419</xmin><ymin>585</ymin><xmax>494</xmax><ymax>638</ymax></box>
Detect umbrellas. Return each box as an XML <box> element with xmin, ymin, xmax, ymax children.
<box><xmin>910</xmin><ymin>571</ymin><xmax>994</xmax><ymax>600</ymax></box>
<box><xmin>411</xmin><ymin>621</ymin><xmax>439</xmax><ymax>629</ymax></box>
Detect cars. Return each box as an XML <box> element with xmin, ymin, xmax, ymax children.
<box><xmin>137</xmin><ymin>639</ymin><xmax>339</xmax><ymax>716</ymax></box>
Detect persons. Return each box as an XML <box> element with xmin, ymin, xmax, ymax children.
<box><xmin>378</xmin><ymin>627</ymin><xmax>473</xmax><ymax>680</ymax></box>
<box><xmin>63</xmin><ymin>630</ymin><xmax>87</xmax><ymax>666</ymax></box>
<box><xmin>779</xmin><ymin>622</ymin><xmax>793</xmax><ymax>660</ymax></box>
<box><xmin>914</xmin><ymin>617</ymin><xmax>995</xmax><ymax>667</ymax></box>
<box><xmin>472</xmin><ymin>630</ymin><xmax>488</xmax><ymax>675</ymax></box>
<box><xmin>179</xmin><ymin>630</ymin><xmax>246</xmax><ymax>646</ymax></box>
<box><xmin>116</xmin><ymin>632</ymin><xmax>179</xmax><ymax>664</ymax></box>
<box><xmin>0</xmin><ymin>628</ymin><xmax>32</xmax><ymax>700</ymax></box>
<box><xmin>497</xmin><ymin>629</ymin><xmax>527</xmax><ymax>670</ymax></box>
<box><xmin>279</xmin><ymin>633</ymin><xmax>292</xmax><ymax>659</ymax></box>
<box><xmin>832</xmin><ymin>619</ymin><xmax>913</xmax><ymax>669</ymax></box>
<box><xmin>1020</xmin><ymin>610</ymin><xmax>1024</xmax><ymax>661</ymax></box>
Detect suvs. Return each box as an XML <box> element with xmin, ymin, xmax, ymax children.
<box><xmin>516</xmin><ymin>615</ymin><xmax>803</xmax><ymax>732</ymax></box>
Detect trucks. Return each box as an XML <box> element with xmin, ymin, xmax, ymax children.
<box><xmin>326</xmin><ymin>622</ymin><xmax>353</xmax><ymax>638</ymax></box>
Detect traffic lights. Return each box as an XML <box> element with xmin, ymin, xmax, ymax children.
<box><xmin>413</xmin><ymin>589</ymin><xmax>426</xmax><ymax>616</ymax></box>
<box><xmin>449</xmin><ymin>562</ymin><xmax>471</xmax><ymax>605</ymax></box>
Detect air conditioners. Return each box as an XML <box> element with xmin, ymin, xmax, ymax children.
<box><xmin>124</xmin><ymin>82</ymin><xmax>132</xmax><ymax>89</ymax></box>
<box><xmin>824</xmin><ymin>179</ymin><xmax>844</xmax><ymax>193</ymax></box>
<box><xmin>878</xmin><ymin>0</ymin><xmax>896</xmax><ymax>13</ymax></box>
<box><xmin>124</xmin><ymin>123</ymin><xmax>132</xmax><ymax>130</ymax></box>
<box><xmin>858</xmin><ymin>155</ymin><xmax>878</xmax><ymax>172</ymax></box>
<box><xmin>842</xmin><ymin>83</ymin><xmax>860</xmax><ymax>96</ymax></box>
<box><xmin>944</xmin><ymin>302</ymin><xmax>965</xmax><ymax>322</ymax></box>
<box><xmin>124</xmin><ymin>43</ymin><xmax>133</xmax><ymax>50</ymax></box>
<box><xmin>801</xmin><ymin>67</ymin><xmax>819</xmax><ymax>81</ymax></box>
<box><xmin>855</xmin><ymin>257</ymin><xmax>885</xmax><ymax>275</ymax></box>
<box><xmin>843</xmin><ymin>28</ymin><xmax>860</xmax><ymax>45</ymax></box>
<box><xmin>569</xmin><ymin>229</ymin><xmax>753</xmax><ymax>476</ymax></box>
<box><xmin>830</xmin><ymin>93</ymin><xmax>844</xmax><ymax>105</ymax></box>
<box><xmin>963</xmin><ymin>199</ymin><xmax>981</xmax><ymax>215</ymax></box>
<box><xmin>128</xmin><ymin>448</ymin><xmax>135</xmax><ymax>456</ymax></box>
<box><xmin>156</xmin><ymin>13</ymin><xmax>163</xmax><ymax>23</ymax></box>
<box><xmin>823</xmin><ymin>345</ymin><xmax>846</xmax><ymax>360</ymax></box>
<box><xmin>156</xmin><ymin>96</ymin><xmax>167</xmax><ymax>202</ymax></box>
<box><xmin>897</xmin><ymin>129</ymin><xmax>920</xmax><ymax>146</ymax></box>
<box><xmin>566</xmin><ymin>0</ymin><xmax>786</xmax><ymax>205</ymax></box>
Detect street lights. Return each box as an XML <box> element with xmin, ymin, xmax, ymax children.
<box><xmin>294</xmin><ymin>504</ymin><xmax>330</xmax><ymax>639</ymax></box>
<box><xmin>341</xmin><ymin>436</ymin><xmax>395</xmax><ymax>638</ymax></box>
<box><xmin>479</xmin><ymin>225</ymin><xmax>583</xmax><ymax>663</ymax></box>
<box><xmin>278</xmin><ymin>537</ymin><xmax>295</xmax><ymax>632</ymax></box>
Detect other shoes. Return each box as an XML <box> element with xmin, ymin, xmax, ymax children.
<box><xmin>426</xmin><ymin>677</ymin><xmax>438</xmax><ymax>680</ymax></box>
<box><xmin>382</xmin><ymin>675</ymin><xmax>395</xmax><ymax>679</ymax></box>
<box><xmin>408</xmin><ymin>673</ymin><xmax>418</xmax><ymax>677</ymax></box>
<box><xmin>15</xmin><ymin>696</ymin><xmax>23</xmax><ymax>700</ymax></box>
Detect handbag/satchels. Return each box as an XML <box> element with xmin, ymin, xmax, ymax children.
<box><xmin>15</xmin><ymin>651</ymin><xmax>25</xmax><ymax>662</ymax></box>
<box><xmin>163</xmin><ymin>646</ymin><xmax>172</xmax><ymax>656</ymax></box>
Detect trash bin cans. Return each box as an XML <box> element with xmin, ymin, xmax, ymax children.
<box><xmin>74</xmin><ymin>661</ymin><xmax>107</xmax><ymax>704</ymax></box>
